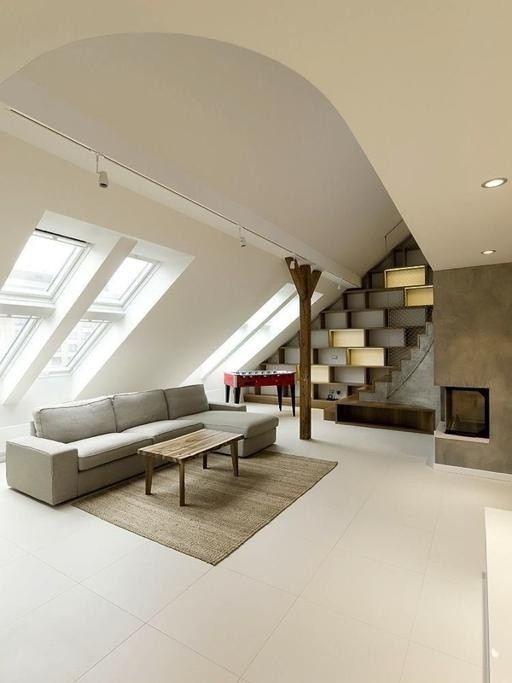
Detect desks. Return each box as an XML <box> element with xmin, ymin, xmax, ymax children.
<box><xmin>223</xmin><ymin>369</ymin><xmax>297</xmax><ymax>417</ymax></box>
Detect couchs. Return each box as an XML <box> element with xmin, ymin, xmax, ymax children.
<box><xmin>4</xmin><ymin>383</ymin><xmax>280</xmax><ymax>509</ymax></box>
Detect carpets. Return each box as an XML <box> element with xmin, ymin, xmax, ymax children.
<box><xmin>70</xmin><ymin>448</ymin><xmax>339</xmax><ymax>567</ymax></box>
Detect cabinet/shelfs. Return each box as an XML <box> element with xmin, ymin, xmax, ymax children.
<box><xmin>254</xmin><ymin>232</ymin><xmax>437</xmax><ymax>434</ymax></box>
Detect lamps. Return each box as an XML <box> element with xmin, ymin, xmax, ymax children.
<box><xmin>238</xmin><ymin>226</ymin><xmax>247</xmax><ymax>249</ymax></box>
<box><xmin>95</xmin><ymin>151</ymin><xmax>108</xmax><ymax>188</ymax></box>
